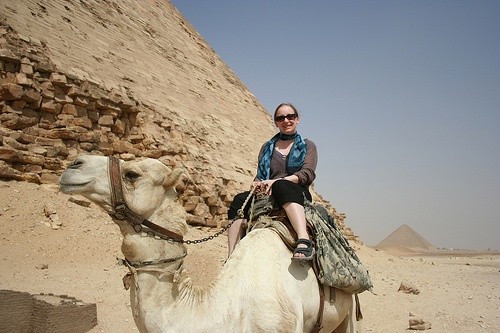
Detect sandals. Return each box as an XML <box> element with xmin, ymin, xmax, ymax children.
<box><xmin>290</xmin><ymin>239</ymin><xmax>315</xmax><ymax>261</ymax></box>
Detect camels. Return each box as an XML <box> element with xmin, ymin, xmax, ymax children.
<box><xmin>59</xmin><ymin>153</ymin><xmax>357</xmax><ymax>333</ymax></box>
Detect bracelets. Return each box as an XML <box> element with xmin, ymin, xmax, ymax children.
<box><xmin>280</xmin><ymin>177</ymin><xmax>286</xmax><ymax>180</ymax></box>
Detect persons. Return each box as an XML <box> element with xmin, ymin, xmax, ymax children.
<box><xmin>227</xmin><ymin>102</ymin><xmax>318</xmax><ymax>261</ymax></box>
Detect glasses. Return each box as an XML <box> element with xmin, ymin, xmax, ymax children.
<box><xmin>275</xmin><ymin>113</ymin><xmax>295</xmax><ymax>122</ymax></box>
